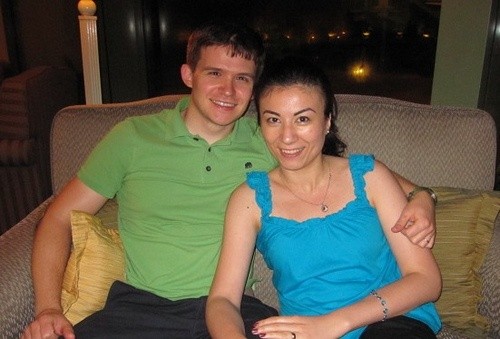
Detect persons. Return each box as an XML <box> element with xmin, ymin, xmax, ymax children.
<box><xmin>18</xmin><ymin>24</ymin><xmax>439</xmax><ymax>339</ymax></box>
<box><xmin>205</xmin><ymin>57</ymin><xmax>444</xmax><ymax>339</ymax></box>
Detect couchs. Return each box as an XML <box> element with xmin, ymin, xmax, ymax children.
<box><xmin>0</xmin><ymin>94</ymin><xmax>500</xmax><ymax>339</ymax></box>
<box><xmin>0</xmin><ymin>65</ymin><xmax>79</xmax><ymax>236</ymax></box>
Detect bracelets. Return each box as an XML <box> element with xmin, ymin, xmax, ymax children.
<box><xmin>370</xmin><ymin>291</ymin><xmax>388</xmax><ymax>322</ymax></box>
<box><xmin>408</xmin><ymin>186</ymin><xmax>438</xmax><ymax>206</ymax></box>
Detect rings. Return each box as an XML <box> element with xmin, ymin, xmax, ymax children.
<box><xmin>290</xmin><ymin>332</ymin><xmax>296</xmax><ymax>339</ymax></box>
<box><xmin>424</xmin><ymin>238</ymin><xmax>429</xmax><ymax>243</ymax></box>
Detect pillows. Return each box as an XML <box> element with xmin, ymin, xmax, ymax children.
<box><xmin>424</xmin><ymin>186</ymin><xmax>499</xmax><ymax>333</ymax></box>
<box><xmin>60</xmin><ymin>211</ymin><xmax>126</xmax><ymax>327</ymax></box>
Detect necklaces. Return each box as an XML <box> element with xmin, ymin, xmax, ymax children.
<box><xmin>279</xmin><ymin>155</ymin><xmax>331</xmax><ymax>213</ymax></box>
